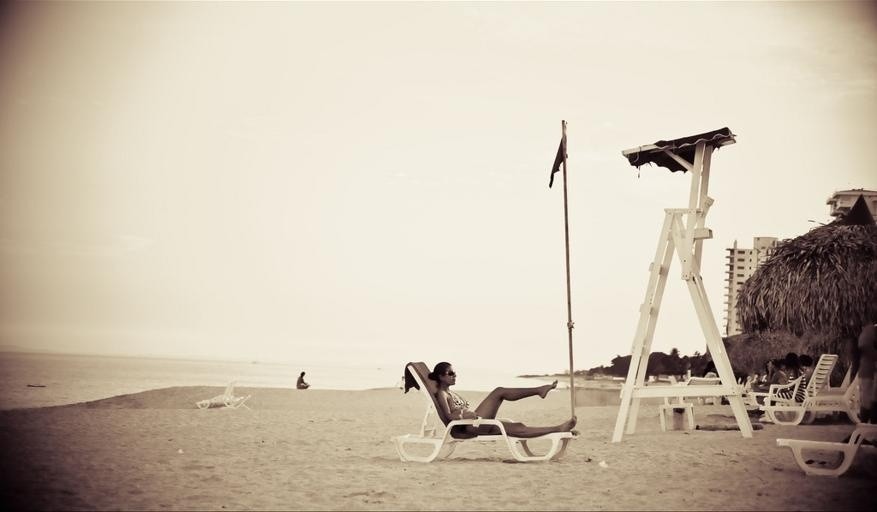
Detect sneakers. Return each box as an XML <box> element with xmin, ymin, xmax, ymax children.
<box><xmin>758</xmin><ymin>414</ymin><xmax>772</xmax><ymax>423</ymax></box>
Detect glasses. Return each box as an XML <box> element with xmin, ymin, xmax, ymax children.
<box><xmin>445</xmin><ymin>370</ymin><xmax>455</xmax><ymax>376</ymax></box>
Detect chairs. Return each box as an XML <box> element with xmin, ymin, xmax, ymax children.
<box><xmin>776</xmin><ymin>422</ymin><xmax>877</xmax><ymax>477</ymax></box>
<box><xmin>195</xmin><ymin>380</ymin><xmax>253</xmax><ymax>411</ymax></box>
<box><xmin>393</xmin><ymin>362</ymin><xmax>573</xmax><ymax>463</ymax></box>
<box><xmin>658</xmin><ymin>354</ymin><xmax>862</xmax><ymax>432</ymax></box>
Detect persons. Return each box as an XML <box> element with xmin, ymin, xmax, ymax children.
<box><xmin>753</xmin><ymin>350</ymin><xmax>806</xmax><ymax>414</ymax></box>
<box><xmin>793</xmin><ymin>353</ymin><xmax>813</xmax><ymax>384</ymax></box>
<box><xmin>296</xmin><ymin>371</ymin><xmax>310</xmax><ymax>389</ymax></box>
<box><xmin>756</xmin><ymin>358</ymin><xmax>788</xmax><ymax>394</ymax></box>
<box><xmin>764</xmin><ymin>360</ymin><xmax>775</xmax><ymax>380</ymax></box>
<box><xmin>425</xmin><ymin>361</ymin><xmax>576</xmax><ymax>439</ymax></box>
<box><xmin>854</xmin><ymin>309</ymin><xmax>876</xmax><ymax>423</ymax></box>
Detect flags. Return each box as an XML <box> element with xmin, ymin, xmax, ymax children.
<box><xmin>548</xmin><ymin>137</ymin><xmax>565</xmax><ymax>190</ymax></box>
